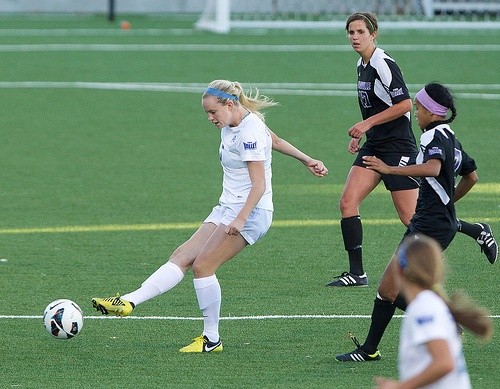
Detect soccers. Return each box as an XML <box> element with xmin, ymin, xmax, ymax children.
<box><xmin>42</xmin><ymin>298</ymin><xmax>84</xmax><ymax>340</ymax></box>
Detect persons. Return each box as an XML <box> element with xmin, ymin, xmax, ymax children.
<box><xmin>335</xmin><ymin>81</ymin><xmax>488</xmax><ymax>363</ymax></box>
<box><xmin>375</xmin><ymin>233</ymin><xmax>490</xmax><ymax>389</ymax></box>
<box><xmin>326</xmin><ymin>13</ymin><xmax>498</xmax><ymax>286</ymax></box>
<box><xmin>91</xmin><ymin>79</ymin><xmax>329</xmax><ymax>352</ymax></box>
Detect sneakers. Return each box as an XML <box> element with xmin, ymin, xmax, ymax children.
<box><xmin>91</xmin><ymin>292</ymin><xmax>136</xmax><ymax>317</ymax></box>
<box><xmin>335</xmin><ymin>336</ymin><xmax>385</xmax><ymax>363</ymax></box>
<box><xmin>179</xmin><ymin>333</ymin><xmax>223</xmax><ymax>353</ymax></box>
<box><xmin>324</xmin><ymin>271</ymin><xmax>369</xmax><ymax>287</ymax></box>
<box><xmin>474</xmin><ymin>222</ymin><xmax>499</xmax><ymax>264</ymax></box>
